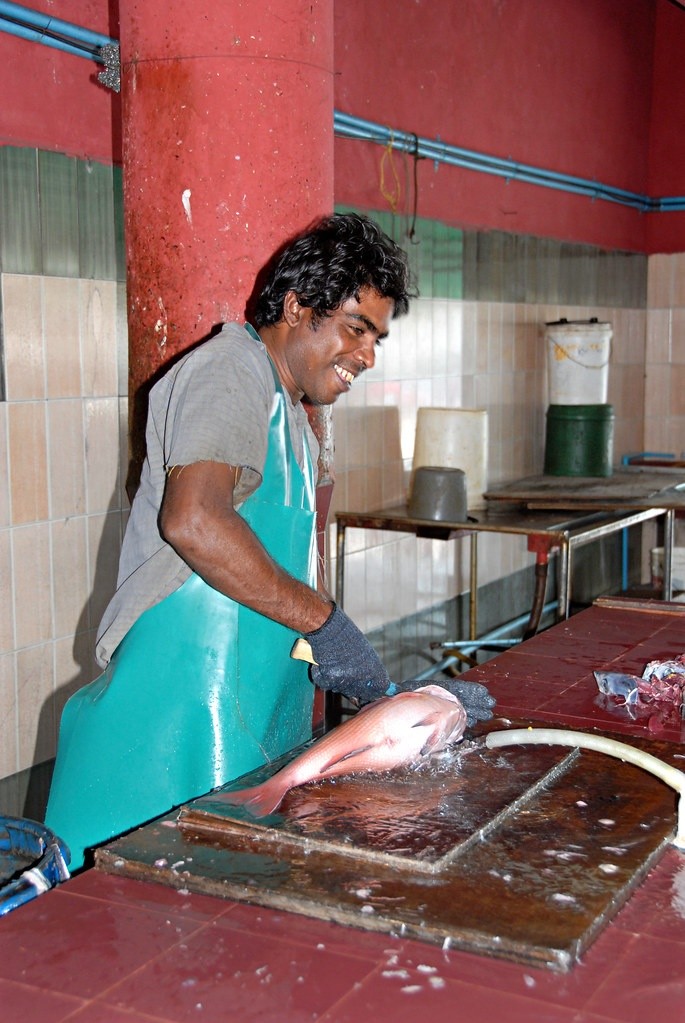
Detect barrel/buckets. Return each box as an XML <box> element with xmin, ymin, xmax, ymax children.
<box><xmin>407</xmin><ymin>466</ymin><xmax>468</xmax><ymax>523</ymax></box>
<box><xmin>545</xmin><ymin>316</ymin><xmax>614</xmax><ymax>405</ymax></box>
<box><xmin>542</xmin><ymin>404</ymin><xmax>615</xmax><ymax>478</ymax></box>
<box><xmin>0</xmin><ymin>814</ymin><xmax>72</xmax><ymax>917</ymax></box>
<box><xmin>652</xmin><ymin>546</ymin><xmax>685</xmax><ymax>590</ymax></box>
<box><xmin>407</xmin><ymin>405</ymin><xmax>489</xmax><ymax>512</ymax></box>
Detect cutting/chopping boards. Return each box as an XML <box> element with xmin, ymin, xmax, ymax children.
<box><xmin>95</xmin><ymin>714</ymin><xmax>685</xmax><ymax>974</ymax></box>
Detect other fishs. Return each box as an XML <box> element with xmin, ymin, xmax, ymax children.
<box><xmin>198</xmin><ymin>685</ymin><xmax>468</xmax><ymax>819</ymax></box>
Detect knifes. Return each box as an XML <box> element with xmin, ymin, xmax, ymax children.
<box><xmin>290</xmin><ymin>638</ymin><xmax>409</xmax><ymax>698</ymax></box>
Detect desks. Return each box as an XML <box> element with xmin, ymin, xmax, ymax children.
<box><xmin>325</xmin><ymin>482</ymin><xmax>674</xmax><ymax>732</ymax></box>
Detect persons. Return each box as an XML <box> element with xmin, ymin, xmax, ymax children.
<box><xmin>37</xmin><ymin>215</ymin><xmax>494</xmax><ymax>878</ymax></box>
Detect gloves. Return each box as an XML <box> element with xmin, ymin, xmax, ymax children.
<box><xmin>306</xmin><ymin>600</ymin><xmax>391</xmax><ymax>705</ymax></box>
<box><xmin>394</xmin><ymin>678</ymin><xmax>496</xmax><ymax>729</ymax></box>
